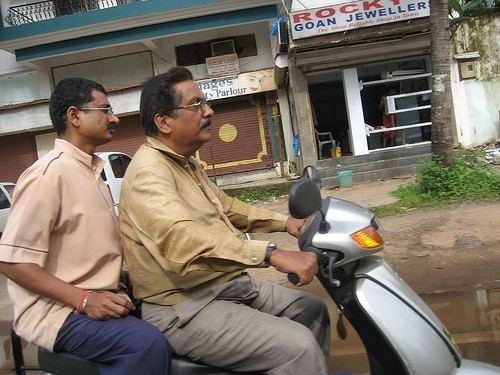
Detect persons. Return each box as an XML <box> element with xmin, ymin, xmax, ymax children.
<box><xmin>381</xmin><ymin>87</ymin><xmax>398</xmax><ymax>147</ymax></box>
<box><xmin>116</xmin><ymin>68</ymin><xmax>330</xmax><ymax>375</ymax></box>
<box><xmin>0</xmin><ymin>78</ymin><xmax>172</xmax><ymax>375</ymax></box>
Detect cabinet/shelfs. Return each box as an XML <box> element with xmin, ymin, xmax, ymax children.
<box><xmin>359</xmin><ymin>73</ymin><xmax>432</xmax><ymax>133</ymax></box>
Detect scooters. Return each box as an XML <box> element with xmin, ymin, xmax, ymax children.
<box><xmin>10</xmin><ymin>165</ymin><xmax>500</xmax><ymax>374</ymax></box>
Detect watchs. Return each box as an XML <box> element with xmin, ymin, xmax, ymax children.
<box><xmin>265</xmin><ymin>242</ymin><xmax>277</xmax><ymax>268</ymax></box>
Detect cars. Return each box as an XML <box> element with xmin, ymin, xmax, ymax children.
<box><xmin>93</xmin><ymin>152</ymin><xmax>133</xmax><ymax>217</ymax></box>
<box><xmin>0</xmin><ymin>182</ymin><xmax>16</xmax><ymax>239</ymax></box>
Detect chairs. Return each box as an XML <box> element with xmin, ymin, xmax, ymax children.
<box><xmin>314</xmin><ymin>129</ymin><xmax>333</xmax><ymax>159</ymax></box>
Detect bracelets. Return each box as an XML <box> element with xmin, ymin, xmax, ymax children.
<box><xmin>75</xmin><ymin>290</ymin><xmax>93</xmax><ymax>313</ymax></box>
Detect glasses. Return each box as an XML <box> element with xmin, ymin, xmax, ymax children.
<box><xmin>167</xmin><ymin>101</ymin><xmax>211</xmax><ymax>112</ymax></box>
<box><xmin>77</xmin><ymin>106</ymin><xmax>113</xmax><ymax>115</ymax></box>
